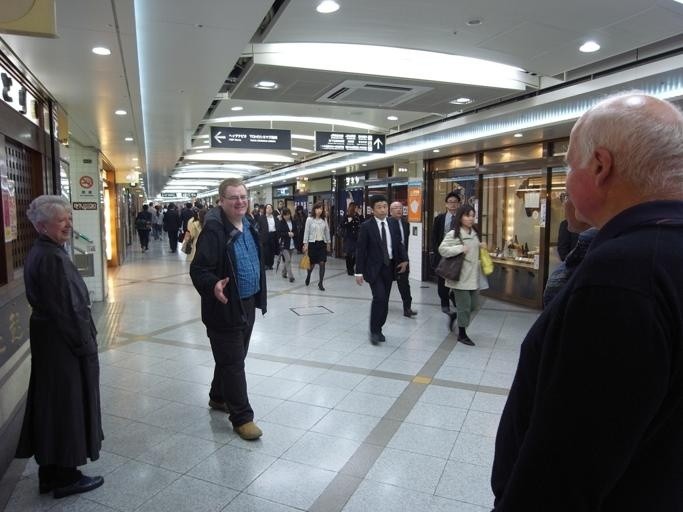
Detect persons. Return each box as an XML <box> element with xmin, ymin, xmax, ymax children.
<box><xmin>489</xmin><ymin>87</ymin><xmax>682</xmax><ymax>512</ymax></box>
<box><xmin>190</xmin><ymin>178</ymin><xmax>267</xmax><ymax>441</ymax></box>
<box><xmin>13</xmin><ymin>195</ymin><xmax>104</xmax><ymax>500</ymax></box>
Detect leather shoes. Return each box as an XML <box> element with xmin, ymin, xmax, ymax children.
<box><xmin>40</xmin><ymin>475</ymin><xmax>103</xmax><ymax>498</ymax></box>
<box><xmin>458</xmin><ymin>335</ymin><xmax>474</xmax><ymax>345</ymax></box>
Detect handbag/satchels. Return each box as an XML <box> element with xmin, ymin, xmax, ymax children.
<box><xmin>435</xmin><ymin>253</ymin><xmax>463</xmax><ymax>280</ymax></box>
<box><xmin>300</xmin><ymin>255</ymin><xmax>310</xmax><ymax>269</ymax></box>
<box><xmin>181</xmin><ymin>242</ymin><xmax>191</xmax><ymax>254</ymax></box>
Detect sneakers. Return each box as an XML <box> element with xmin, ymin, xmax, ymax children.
<box><xmin>209</xmin><ymin>400</ymin><xmax>262</xmax><ymax>440</ymax></box>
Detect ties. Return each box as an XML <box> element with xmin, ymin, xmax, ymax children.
<box><xmin>381</xmin><ymin>222</ymin><xmax>389</xmax><ymax>265</ymax></box>
